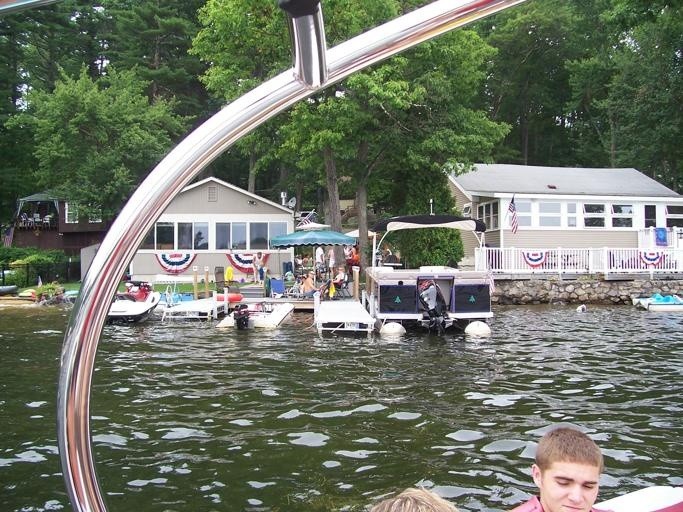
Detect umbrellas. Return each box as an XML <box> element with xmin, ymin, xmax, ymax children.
<box><xmin>270</xmin><ymin>229</ymin><xmax>359</xmax><ymax>288</ymax></box>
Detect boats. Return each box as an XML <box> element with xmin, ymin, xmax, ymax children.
<box><xmin>58</xmin><ymin>2</ymin><xmax>683</xmax><ymax>511</ymax></box>
<box><xmin>107</xmin><ymin>278</ymin><xmax>164</xmax><ymax>327</ymax></box>
<box><xmin>360</xmin><ymin>198</ymin><xmax>499</xmax><ymax>344</ymax></box>
<box><xmin>637</xmin><ymin>291</ymin><xmax>683</xmax><ymax>313</ymax></box>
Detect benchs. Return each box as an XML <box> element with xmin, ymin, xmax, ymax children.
<box><xmin>284</xmin><ymin>292</ymin><xmax>315</xmax><ymax>300</ymax></box>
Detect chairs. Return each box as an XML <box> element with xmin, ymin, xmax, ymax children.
<box><xmin>328</xmin><ymin>274</ymin><xmax>351</xmax><ymax>299</ymax></box>
<box><xmin>270</xmin><ymin>278</ymin><xmax>289</xmax><ymax>298</ymax></box>
<box><xmin>294</xmin><ymin>259</ymin><xmax>304</xmax><ymax>278</ymax></box>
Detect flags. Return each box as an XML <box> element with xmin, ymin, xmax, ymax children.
<box><xmin>508</xmin><ymin>196</ymin><xmax>518</xmax><ymax>234</ymax></box>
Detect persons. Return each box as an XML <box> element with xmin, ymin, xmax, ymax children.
<box><xmin>252</xmin><ymin>252</ymin><xmax>264</xmax><ymax>284</ymax></box>
<box><xmin>294</xmin><ymin>245</ymin><xmax>360</xmax><ymax>300</ymax></box>
<box><xmin>508</xmin><ymin>428</ymin><xmax>604</xmax><ymax>512</ymax></box>
<box><xmin>371</xmin><ymin>486</ymin><xmax>458</xmax><ymax>512</ymax></box>
